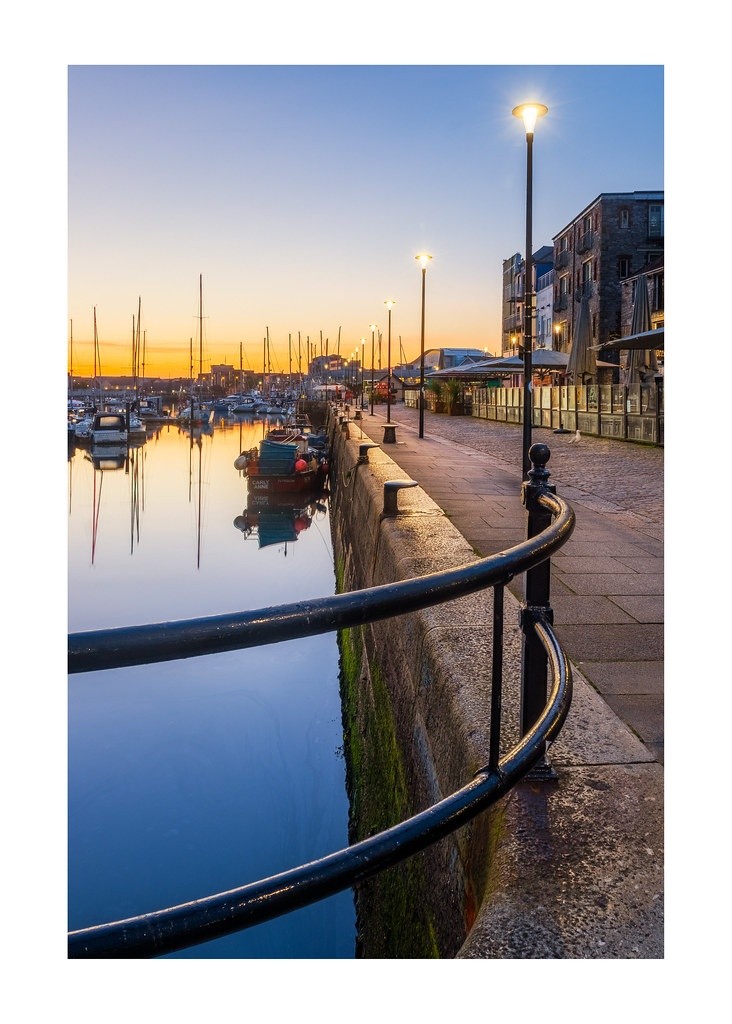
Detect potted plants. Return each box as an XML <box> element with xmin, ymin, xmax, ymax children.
<box><xmin>430</xmin><ymin>379</ymin><xmax>447</xmax><ymax>413</ymax></box>
<box><xmin>445</xmin><ymin>378</ymin><xmax>464</xmax><ymax>415</ymax></box>
<box><xmin>368</xmin><ymin>391</ymin><xmax>382</xmax><ymax>404</ymax></box>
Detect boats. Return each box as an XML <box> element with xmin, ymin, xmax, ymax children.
<box><xmin>266</xmin><ymin>422</ymin><xmax>327</xmax><ymax>451</ymax></box>
<box><xmin>233</xmin><ymin>493</ymin><xmax>328</xmax><ymax>556</ymax></box>
<box><xmin>233</xmin><ymin>430</ymin><xmax>328</xmax><ymax>493</ymax></box>
<box><xmin>68</xmin><ymin>273</ymin><xmax>295</xmax><ymax>445</ymax></box>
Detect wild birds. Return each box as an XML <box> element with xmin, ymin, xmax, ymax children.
<box><xmin>569</xmin><ymin>430</ymin><xmax>581</xmax><ymax>445</ymax></box>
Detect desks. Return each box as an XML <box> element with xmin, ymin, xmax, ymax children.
<box><xmin>355</xmin><ymin>410</ymin><xmax>362</xmax><ymax>419</ymax></box>
<box><xmin>381</xmin><ymin>425</ymin><xmax>399</xmax><ymax>443</ymax></box>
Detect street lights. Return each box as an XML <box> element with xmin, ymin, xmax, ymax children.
<box><xmin>344</xmin><ymin>352</ymin><xmax>355</xmax><ymax>405</ymax></box>
<box><xmin>369</xmin><ymin>324</ymin><xmax>378</xmax><ymax>416</ymax></box>
<box><xmin>414</xmin><ymin>253</ymin><xmax>432</xmax><ymax>439</ymax></box>
<box><xmin>354</xmin><ymin>347</ymin><xmax>360</xmax><ymax>409</ymax></box>
<box><xmin>361</xmin><ymin>338</ymin><xmax>368</xmax><ymax>412</ymax></box>
<box><xmin>513</xmin><ymin>100</ymin><xmax>548</xmax><ymax>510</ymax></box>
<box><xmin>384</xmin><ymin>300</ymin><xmax>396</xmax><ymax>424</ymax></box>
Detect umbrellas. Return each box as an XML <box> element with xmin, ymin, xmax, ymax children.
<box><xmin>566</xmin><ymin>295</ymin><xmax>599</xmax><ymax>385</ymax></box>
<box><xmin>626</xmin><ymin>274</ymin><xmax>659</xmax><ymax>381</ymax></box>
<box><xmin>588</xmin><ymin>327</ymin><xmax>664</xmax><ymax>350</ymax></box>
<box><xmin>423</xmin><ymin>348</ymin><xmax>625</xmax><ymax>386</ymax></box>
